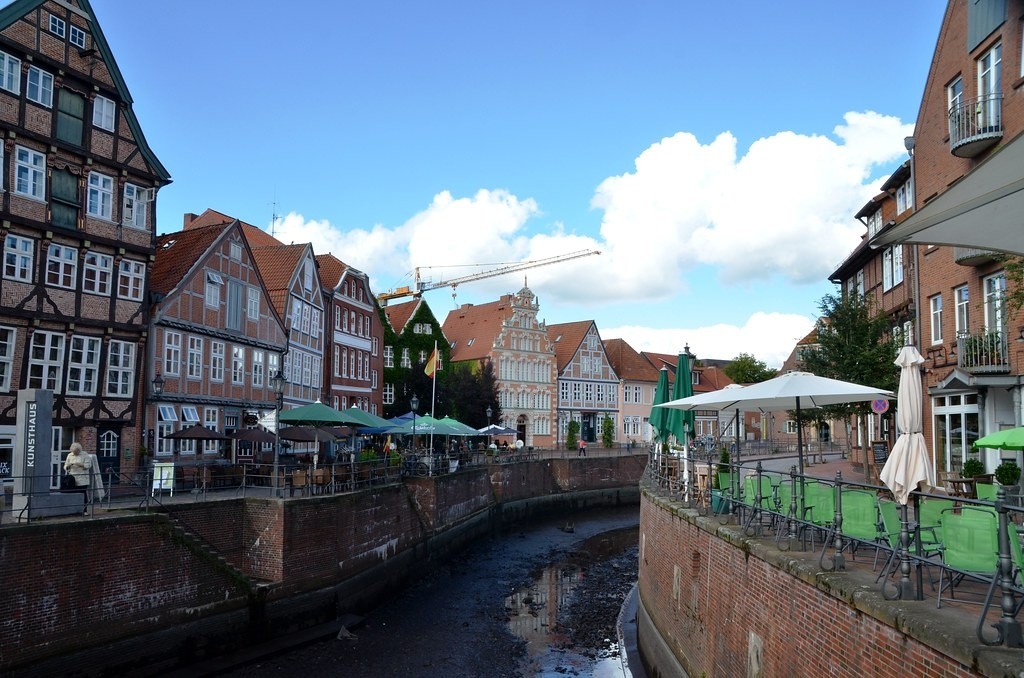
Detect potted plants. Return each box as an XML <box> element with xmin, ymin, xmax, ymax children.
<box><xmin>996</xmin><ymin>463</ymin><xmax>1021</xmax><ymax>507</ymax></box>
<box><xmin>960</xmin><ymin>459</ymin><xmax>984</xmax><ymax>492</ymax></box>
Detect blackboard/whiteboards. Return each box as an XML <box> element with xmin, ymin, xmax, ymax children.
<box><xmin>870</xmin><ymin>440</ymin><xmax>889</xmax><ymax>464</ymax></box>
<box><xmin>153</xmin><ymin>463</ymin><xmax>176</xmax><ymax>490</ymax></box>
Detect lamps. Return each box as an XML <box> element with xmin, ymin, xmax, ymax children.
<box><xmin>925</xmin><ymin>342</ymin><xmax>957</xmax><ymax>362</ymax></box>
<box><xmin>1016</xmin><ymin>328</ymin><xmax>1024</xmax><ymax>342</ymax></box>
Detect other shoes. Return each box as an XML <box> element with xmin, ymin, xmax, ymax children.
<box><xmin>83</xmin><ymin>512</ymin><xmax>88</xmax><ymax>516</ymax></box>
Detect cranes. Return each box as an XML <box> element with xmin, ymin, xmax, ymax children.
<box><xmin>375</xmin><ymin>248</ymin><xmax>601</xmax><ymax>308</ymax></box>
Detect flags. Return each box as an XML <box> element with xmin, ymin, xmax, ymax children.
<box><xmin>424</xmin><ymin>347</ymin><xmax>439</xmax><ymax>378</ymax></box>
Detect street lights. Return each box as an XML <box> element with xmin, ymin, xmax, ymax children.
<box><xmin>410</xmin><ymin>394</ymin><xmax>419</xmax><ymax>473</ymax></box>
<box><xmin>152</xmin><ymin>370</ymin><xmax>167</xmax><ymax>464</ymax></box>
<box><xmin>270</xmin><ymin>367</ymin><xmax>288</xmax><ymax>497</ymax></box>
<box><xmin>486</xmin><ymin>405</ymin><xmax>493</xmax><ymax>449</ymax></box>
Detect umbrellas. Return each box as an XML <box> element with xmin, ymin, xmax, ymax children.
<box><xmin>648</xmin><ymin>351</ymin><xmax>897</xmax><ymax>552</ymax></box>
<box><xmin>162</xmin><ymin>398</ymin><xmax>522</xmax><ymax>492</ymax></box>
<box><xmin>879</xmin><ymin>346</ymin><xmax>936</xmax><ymax>601</ymax></box>
<box><xmin>972</xmin><ymin>427</ymin><xmax>1024</xmax><ymax>477</ymax></box>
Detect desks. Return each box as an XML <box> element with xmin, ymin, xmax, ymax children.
<box><xmin>943</xmin><ymin>478</ymin><xmax>974</xmax><ymax>512</ymax></box>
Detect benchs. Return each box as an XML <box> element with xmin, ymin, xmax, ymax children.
<box><xmin>507</xmin><ymin>453</ymin><xmax>537</xmax><ymax>461</ymax></box>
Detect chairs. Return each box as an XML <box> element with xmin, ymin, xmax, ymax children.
<box><xmin>174</xmin><ymin>463</ymin><xmax>385</xmax><ymax>497</ymax></box>
<box><xmin>654</xmin><ymin>452</ymin><xmax>1024</xmax><ymax>617</ymax></box>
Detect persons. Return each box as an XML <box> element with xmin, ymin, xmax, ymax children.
<box><xmin>396</xmin><ymin>437</ymin><xmax>586</xmax><ymax>457</ymax></box>
<box><xmin>626</xmin><ymin>436</ymin><xmax>631</xmax><ymax>452</ymax></box>
<box><xmin>64</xmin><ymin>444</ymin><xmax>92</xmax><ymax>515</ymax></box>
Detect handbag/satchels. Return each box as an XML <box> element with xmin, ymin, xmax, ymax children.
<box><xmin>583</xmin><ymin>442</ymin><xmax>587</xmax><ymax>448</ymax></box>
<box><xmin>59</xmin><ymin>466</ymin><xmax>76</xmax><ymax>493</ymax></box>
